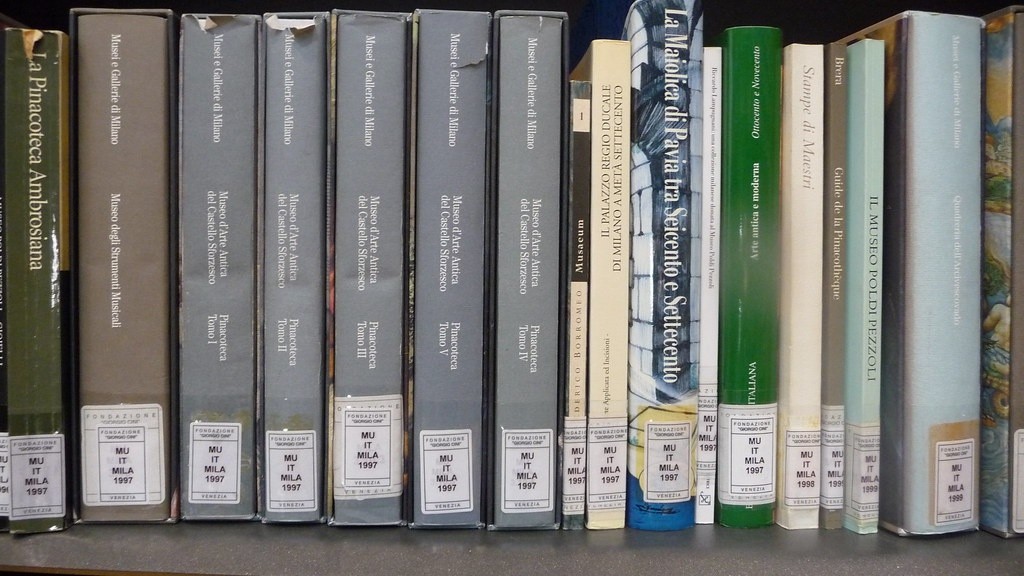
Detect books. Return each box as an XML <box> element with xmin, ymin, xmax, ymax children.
<box><xmin>562</xmin><ymin>78</ymin><xmax>588</xmax><ymax>530</ymax></box>
<box><xmin>823</xmin><ymin>45</ymin><xmax>850</xmax><ymax>529</ymax></box>
<box><xmin>414</xmin><ymin>11</ymin><xmax>489</xmax><ymax>532</ymax></box>
<box><xmin>846</xmin><ymin>35</ymin><xmax>888</xmax><ymax>535</ymax></box>
<box><xmin>622</xmin><ymin>0</ymin><xmax>702</xmax><ymax>530</ymax></box>
<box><xmin>836</xmin><ymin>6</ymin><xmax>986</xmax><ymax>540</ymax></box>
<box><xmin>779</xmin><ymin>40</ymin><xmax>819</xmax><ymax>531</ymax></box>
<box><xmin>981</xmin><ymin>6</ymin><xmax>1024</xmax><ymax>540</ymax></box>
<box><xmin>253</xmin><ymin>12</ymin><xmax>331</xmax><ymax>526</ymax></box>
<box><xmin>0</xmin><ymin>167</ymin><xmax>11</xmax><ymax>532</ymax></box>
<box><xmin>327</xmin><ymin>3</ymin><xmax>411</xmax><ymax>532</ymax></box>
<box><xmin>175</xmin><ymin>8</ymin><xmax>265</xmax><ymax>523</ymax></box>
<box><xmin>693</xmin><ymin>46</ymin><xmax>721</xmax><ymax>527</ymax></box>
<box><xmin>492</xmin><ymin>10</ymin><xmax>569</xmax><ymax>535</ymax></box>
<box><xmin>573</xmin><ymin>35</ymin><xmax>632</xmax><ymax>532</ymax></box>
<box><xmin>6</xmin><ymin>23</ymin><xmax>71</xmax><ymax>539</ymax></box>
<box><xmin>719</xmin><ymin>17</ymin><xmax>779</xmax><ymax>527</ymax></box>
<box><xmin>70</xmin><ymin>3</ymin><xmax>179</xmax><ymax>528</ymax></box>
